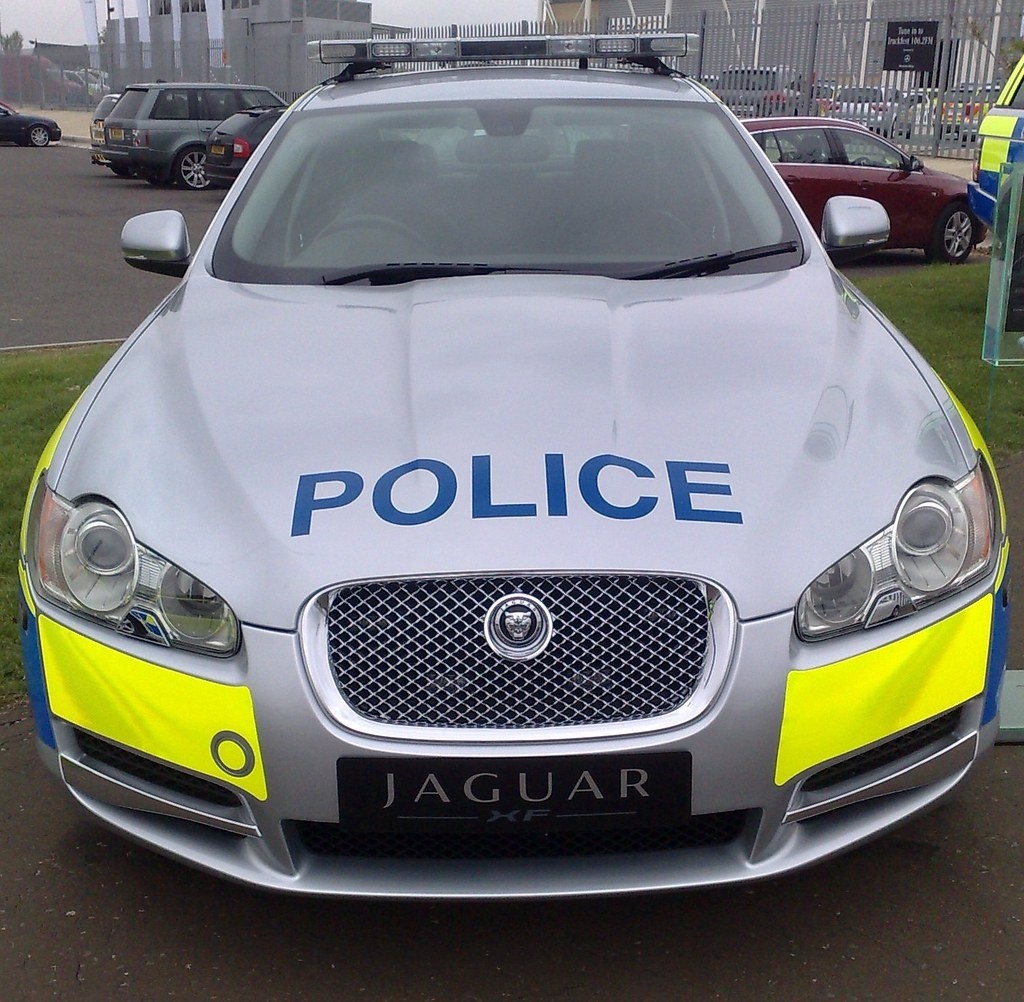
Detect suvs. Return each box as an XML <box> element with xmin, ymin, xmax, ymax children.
<box><xmin>203</xmin><ymin>104</ymin><xmax>292</xmax><ymax>188</ymax></box>
<box><xmin>90</xmin><ymin>93</ymin><xmax>224</xmax><ymax>178</ymax></box>
<box><xmin>102</xmin><ymin>78</ymin><xmax>291</xmax><ymax>192</ymax></box>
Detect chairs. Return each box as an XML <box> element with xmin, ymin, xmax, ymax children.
<box><xmin>439</xmin><ymin>160</ymin><xmax>577</xmax><ymax>245</ymax></box>
<box><xmin>340</xmin><ymin>136</ymin><xmax>438</xmax><ymax>233</ymax></box>
<box><xmin>571</xmin><ymin>138</ymin><xmax>701</xmax><ymax>221</ymax></box>
<box><xmin>797</xmin><ymin>136</ymin><xmax>822</xmax><ymax>163</ymax></box>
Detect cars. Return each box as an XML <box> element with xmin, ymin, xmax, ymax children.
<box><xmin>46</xmin><ymin>66</ymin><xmax>109</xmax><ymax>97</ymax></box>
<box><xmin>687</xmin><ymin>64</ymin><xmax>1003</xmax><ymax>147</ymax></box>
<box><xmin>18</xmin><ymin>34</ymin><xmax>1013</xmax><ymax>899</ymax></box>
<box><xmin>967</xmin><ymin>53</ymin><xmax>1024</xmax><ymax>234</ymax></box>
<box><xmin>739</xmin><ymin>115</ymin><xmax>988</xmax><ymax>265</ymax></box>
<box><xmin>0</xmin><ymin>100</ymin><xmax>62</xmax><ymax>148</ymax></box>
